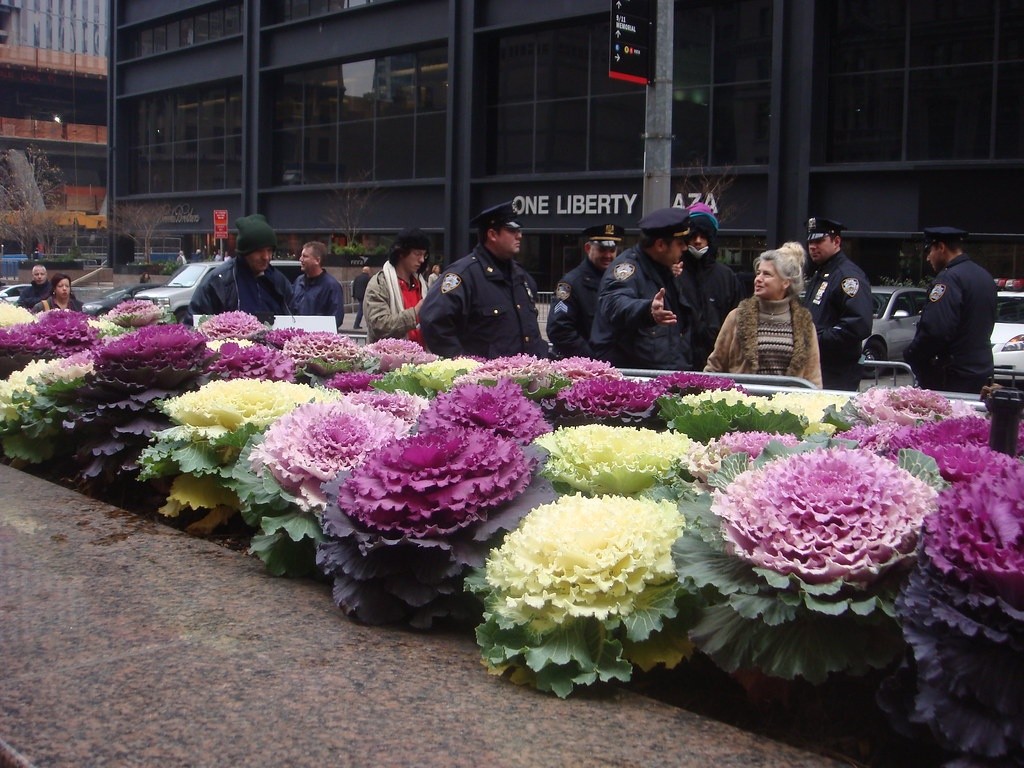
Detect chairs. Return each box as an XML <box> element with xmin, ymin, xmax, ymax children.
<box><xmin>891</xmin><ymin>298</ymin><xmax>911</xmax><ymax>314</ymax></box>
<box><xmin>999</xmin><ymin>302</ymin><xmax>1024</xmax><ymax>321</ymax></box>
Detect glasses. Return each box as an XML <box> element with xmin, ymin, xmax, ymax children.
<box><xmin>598</xmin><ymin>247</ymin><xmax>617</xmax><ymax>253</ymax></box>
<box><xmin>415</xmin><ymin>251</ymin><xmax>428</xmax><ymax>259</ymax></box>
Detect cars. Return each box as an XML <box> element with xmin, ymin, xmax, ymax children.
<box><xmin>0</xmin><ymin>283</ymin><xmax>32</xmax><ymax>304</ymax></box>
<box><xmin>990</xmin><ymin>279</ymin><xmax>1024</xmax><ymax>382</ymax></box>
<box><xmin>81</xmin><ymin>282</ymin><xmax>162</xmax><ymax>318</ymax></box>
<box><xmin>862</xmin><ymin>285</ymin><xmax>926</xmax><ymax>378</ymax></box>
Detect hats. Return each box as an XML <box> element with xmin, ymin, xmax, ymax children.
<box><xmin>234</xmin><ymin>214</ymin><xmax>277</xmax><ymax>256</ymax></box>
<box><xmin>804</xmin><ymin>216</ymin><xmax>847</xmax><ymax>241</ymax></box>
<box><xmin>923</xmin><ymin>227</ymin><xmax>969</xmax><ymax>251</ymax></box>
<box><xmin>638</xmin><ymin>207</ymin><xmax>690</xmax><ymax>239</ymax></box>
<box><xmin>470</xmin><ymin>200</ymin><xmax>523</xmax><ymax>229</ymax></box>
<box><xmin>582</xmin><ymin>224</ymin><xmax>624</xmax><ymax>248</ymax></box>
<box><xmin>684</xmin><ymin>202</ymin><xmax>719</xmax><ymax>232</ymax></box>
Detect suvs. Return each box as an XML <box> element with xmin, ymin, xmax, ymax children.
<box><xmin>131</xmin><ymin>259</ymin><xmax>303</xmax><ymax>327</ymax></box>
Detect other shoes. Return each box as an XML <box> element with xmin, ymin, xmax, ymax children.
<box><xmin>354</xmin><ymin>327</ymin><xmax>362</xmax><ymax>329</ymax></box>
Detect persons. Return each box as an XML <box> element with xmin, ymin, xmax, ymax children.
<box><xmin>176</xmin><ymin>245</ymin><xmax>233</xmax><ymax>264</ymax></box>
<box><xmin>184</xmin><ymin>214</ymin><xmax>292</xmax><ymax>327</ymax></box>
<box><xmin>546</xmin><ymin>202</ymin><xmax>742</xmax><ymax>372</ymax></box>
<box><xmin>703</xmin><ymin>242</ymin><xmax>824</xmax><ymax>389</ymax></box>
<box><xmin>17</xmin><ymin>265</ymin><xmax>83</xmax><ymax>316</ymax></box>
<box><xmin>418</xmin><ymin>200</ymin><xmax>551</xmax><ymax>360</ymax></box>
<box><xmin>902</xmin><ymin>227</ymin><xmax>998</xmax><ymax>394</ymax></box>
<box><xmin>139</xmin><ymin>272</ymin><xmax>152</xmax><ymax>283</ymax></box>
<box><xmin>354</xmin><ymin>261</ymin><xmax>442</xmax><ymax>330</ymax></box>
<box><xmin>802</xmin><ymin>217</ymin><xmax>873</xmax><ymax>390</ymax></box>
<box><xmin>292</xmin><ymin>241</ymin><xmax>344</xmax><ymax>330</ymax></box>
<box><xmin>364</xmin><ymin>229</ymin><xmax>430</xmax><ymax>351</ymax></box>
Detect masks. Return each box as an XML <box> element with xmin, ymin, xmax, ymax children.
<box><xmin>688</xmin><ymin>245</ymin><xmax>709</xmax><ymax>259</ymax></box>
